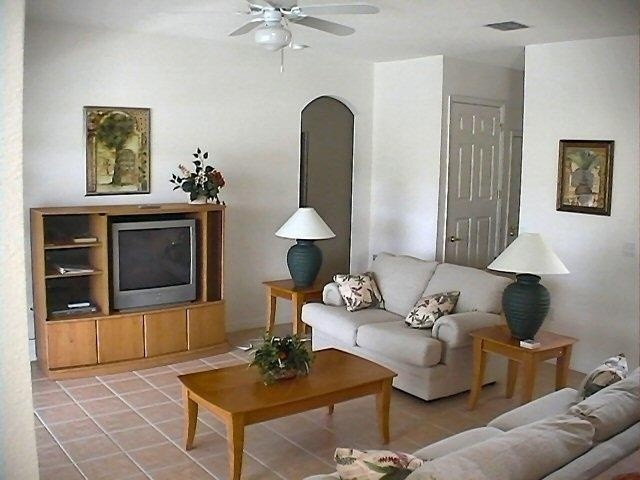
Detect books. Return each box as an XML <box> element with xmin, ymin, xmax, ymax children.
<box><xmin>54</xmin><ymin>260</ymin><xmax>93</xmax><ymax>274</ymax></box>
<box><xmin>60</xmin><ymin>289</ymin><xmax>91</xmax><ymax>308</ymax></box>
<box><xmin>70</xmin><ymin>230</ymin><xmax>99</xmax><ymax>243</ymax></box>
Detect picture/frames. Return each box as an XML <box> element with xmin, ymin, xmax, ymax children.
<box><xmin>82</xmin><ymin>105</ymin><xmax>150</xmax><ymax>199</ymax></box>
<box><xmin>553</xmin><ymin>136</ymin><xmax>616</xmax><ymax>218</ymax></box>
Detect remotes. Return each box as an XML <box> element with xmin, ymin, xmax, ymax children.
<box><xmin>137</xmin><ymin>203</ymin><xmax>161</xmax><ymax>209</ymax></box>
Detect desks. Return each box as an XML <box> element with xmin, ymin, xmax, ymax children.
<box><xmin>177</xmin><ymin>346</ymin><xmax>395</xmax><ymax>478</ymax></box>
<box><xmin>262</xmin><ymin>276</ymin><xmax>332</xmax><ymax>335</ymax></box>
<box><xmin>471</xmin><ymin>324</ymin><xmax>575</xmax><ymax>402</ymax></box>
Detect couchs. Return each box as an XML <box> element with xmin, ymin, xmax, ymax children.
<box><xmin>303</xmin><ymin>365</ymin><xmax>640</xmax><ymax>479</ymax></box>
<box><xmin>302</xmin><ymin>251</ymin><xmax>514</xmax><ymax>401</ymax></box>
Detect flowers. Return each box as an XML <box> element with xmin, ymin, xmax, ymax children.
<box><xmin>244</xmin><ymin>331</ymin><xmax>312</xmax><ymax>378</ymax></box>
<box><xmin>169</xmin><ymin>147</ymin><xmax>225</xmax><ymax>193</ymax></box>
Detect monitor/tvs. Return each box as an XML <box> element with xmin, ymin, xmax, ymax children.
<box><xmin>109</xmin><ymin>219</ymin><xmax>197</xmax><ymax>314</ymax></box>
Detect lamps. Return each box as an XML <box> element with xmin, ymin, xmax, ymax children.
<box><xmin>251</xmin><ymin>12</ymin><xmax>288</xmax><ymax>55</ymax></box>
<box><xmin>488</xmin><ymin>234</ymin><xmax>571</xmax><ymax>341</ymax></box>
<box><xmin>272</xmin><ymin>205</ymin><xmax>336</xmax><ymax>288</ymax></box>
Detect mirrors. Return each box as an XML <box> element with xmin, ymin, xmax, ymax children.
<box><xmin>298</xmin><ymin>93</ymin><xmax>355</xmax><ymax>276</ymax></box>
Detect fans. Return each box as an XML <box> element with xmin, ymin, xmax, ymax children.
<box><xmin>230</xmin><ymin>0</ymin><xmax>378</xmax><ymax>41</ymax></box>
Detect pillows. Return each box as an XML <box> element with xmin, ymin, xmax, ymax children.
<box><xmin>337</xmin><ymin>445</ymin><xmax>421</xmax><ymax>480</ymax></box>
<box><xmin>405</xmin><ymin>292</ymin><xmax>459</xmax><ymax>328</ymax></box>
<box><xmin>332</xmin><ymin>267</ymin><xmax>386</xmax><ymax>312</ymax></box>
<box><xmin>576</xmin><ymin>354</ymin><xmax>629</xmax><ymax>403</ymax></box>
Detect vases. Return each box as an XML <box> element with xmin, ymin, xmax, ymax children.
<box><xmin>189</xmin><ymin>194</ymin><xmax>208</xmax><ymax>203</ymax></box>
<box><xmin>269</xmin><ymin>369</ymin><xmax>300</xmax><ymax>382</ymax></box>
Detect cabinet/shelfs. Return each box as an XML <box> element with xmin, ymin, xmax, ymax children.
<box><xmin>29</xmin><ymin>202</ymin><xmax>232</xmax><ymax>385</ymax></box>
